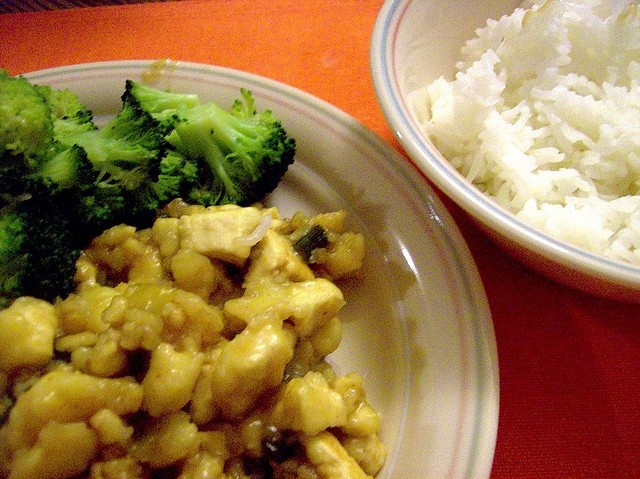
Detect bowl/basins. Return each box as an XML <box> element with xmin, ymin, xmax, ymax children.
<box><xmin>369</xmin><ymin>2</ymin><xmax>640</xmax><ymax>316</ymax></box>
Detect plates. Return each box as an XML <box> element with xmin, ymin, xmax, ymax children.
<box><xmin>1</xmin><ymin>59</ymin><xmax>502</xmax><ymax>479</ymax></box>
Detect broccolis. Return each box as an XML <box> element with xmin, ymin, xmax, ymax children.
<box><xmin>0</xmin><ymin>143</ymin><xmax>212</xmax><ymax>310</ymax></box>
<box><xmin>122</xmin><ymin>80</ymin><xmax>201</xmax><ymax>131</ymax></box>
<box><xmin>0</xmin><ymin>67</ymin><xmax>55</xmax><ymax>167</ymax></box>
<box><xmin>173</xmin><ymin>88</ymin><xmax>296</xmax><ymax>207</ymax></box>
<box><xmin>33</xmin><ymin>82</ymin><xmax>100</xmax><ymax>135</ymax></box>
<box><xmin>60</xmin><ymin>95</ymin><xmax>168</xmax><ymax>191</ymax></box>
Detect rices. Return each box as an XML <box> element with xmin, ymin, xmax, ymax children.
<box><xmin>410</xmin><ymin>2</ymin><xmax>639</xmax><ymax>272</ymax></box>
